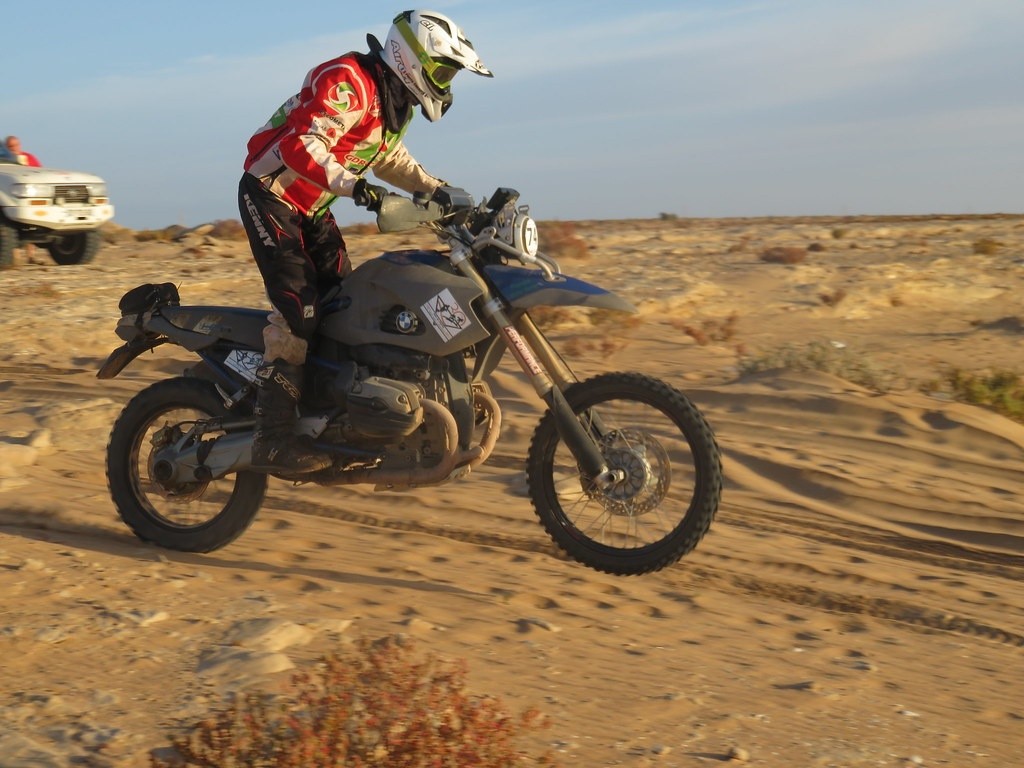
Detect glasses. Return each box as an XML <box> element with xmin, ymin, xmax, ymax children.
<box><xmin>393</xmin><ymin>13</ymin><xmax>459</xmax><ymax>89</ymax></box>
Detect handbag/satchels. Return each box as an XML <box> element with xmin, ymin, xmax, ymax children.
<box><xmin>119</xmin><ymin>282</ymin><xmax>181</xmax><ymax>317</ymax></box>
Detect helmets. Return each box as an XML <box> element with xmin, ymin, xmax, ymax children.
<box><xmin>378</xmin><ymin>9</ymin><xmax>494</xmax><ymax>122</ymax></box>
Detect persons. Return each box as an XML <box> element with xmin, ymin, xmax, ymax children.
<box><xmin>6</xmin><ymin>137</ymin><xmax>46</xmax><ymax>264</ymax></box>
<box><xmin>238</xmin><ymin>11</ymin><xmax>493</xmax><ymax>472</ymax></box>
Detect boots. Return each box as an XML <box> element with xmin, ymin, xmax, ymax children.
<box><xmin>249</xmin><ymin>357</ymin><xmax>333</xmax><ymax>475</ymax></box>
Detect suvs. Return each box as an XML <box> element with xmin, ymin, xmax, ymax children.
<box><xmin>0</xmin><ymin>141</ymin><xmax>115</xmax><ymax>270</ymax></box>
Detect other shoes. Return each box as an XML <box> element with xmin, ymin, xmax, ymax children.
<box><xmin>29</xmin><ymin>257</ymin><xmax>46</xmax><ymax>264</ymax></box>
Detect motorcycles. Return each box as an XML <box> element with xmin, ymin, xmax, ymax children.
<box><xmin>96</xmin><ymin>189</ymin><xmax>725</xmax><ymax>577</ymax></box>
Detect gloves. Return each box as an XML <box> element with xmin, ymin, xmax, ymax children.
<box><xmin>353</xmin><ymin>179</ymin><xmax>388</xmax><ymax>211</ymax></box>
<box><xmin>432</xmin><ymin>181</ymin><xmax>452</xmax><ymax>196</ymax></box>
